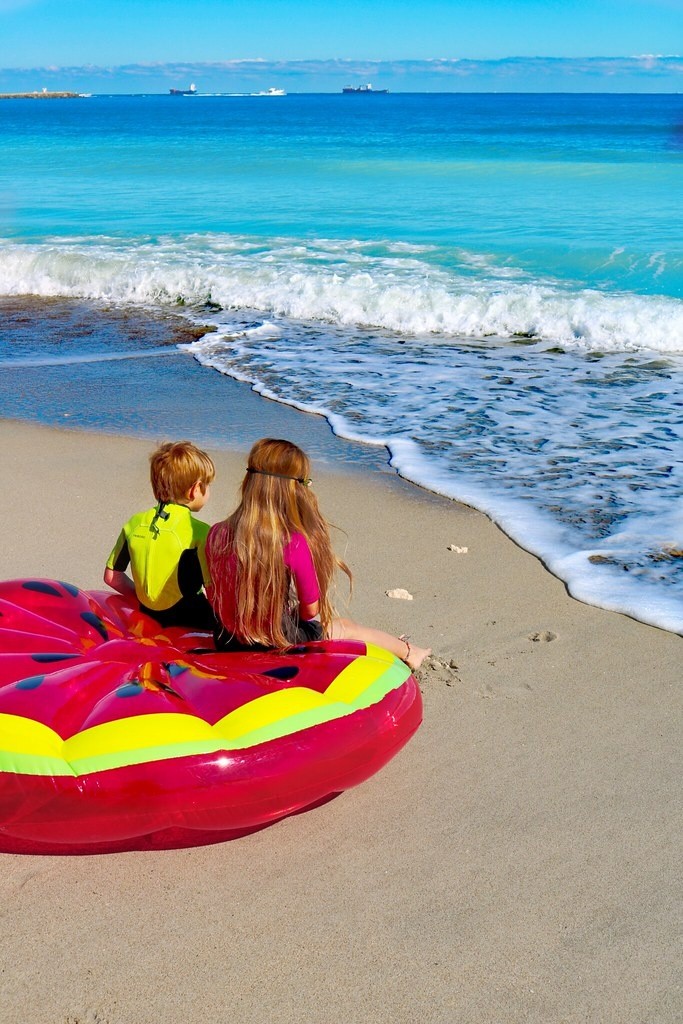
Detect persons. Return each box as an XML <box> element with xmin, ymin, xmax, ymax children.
<box><xmin>205</xmin><ymin>437</ymin><xmax>431</xmax><ymax>671</ymax></box>
<box><xmin>104</xmin><ymin>442</ymin><xmax>223</xmax><ymax>627</ymax></box>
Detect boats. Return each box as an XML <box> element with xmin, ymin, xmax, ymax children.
<box><xmin>168</xmin><ymin>83</ymin><xmax>197</xmax><ymax>94</ymax></box>
<box><xmin>342</xmin><ymin>80</ymin><xmax>390</xmax><ymax>94</ymax></box>
<box><xmin>260</xmin><ymin>87</ymin><xmax>285</xmax><ymax>95</ymax></box>
